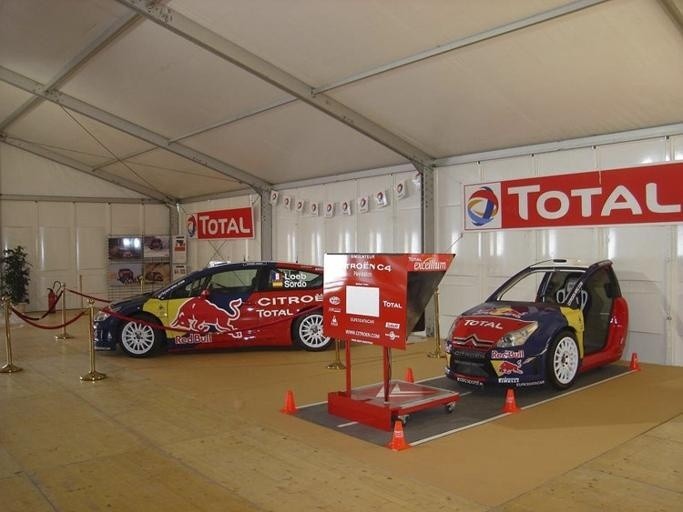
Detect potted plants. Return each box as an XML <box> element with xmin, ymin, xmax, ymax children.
<box><xmin>0</xmin><ymin>245</ymin><xmax>34</xmax><ymax>316</ymax></box>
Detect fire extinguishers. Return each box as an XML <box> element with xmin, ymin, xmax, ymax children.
<box><xmin>46</xmin><ymin>287</ymin><xmax>56</xmax><ymax>314</ymax></box>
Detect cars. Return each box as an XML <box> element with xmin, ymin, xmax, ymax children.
<box><xmin>441</xmin><ymin>256</ymin><xmax>630</xmax><ymax>393</ymax></box>
<box><xmin>90</xmin><ymin>261</ymin><xmax>340</xmax><ymax>360</ymax></box>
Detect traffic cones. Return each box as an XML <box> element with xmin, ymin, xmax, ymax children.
<box><xmin>500</xmin><ymin>387</ymin><xmax>521</xmax><ymax>414</ymax></box>
<box><xmin>404</xmin><ymin>367</ymin><xmax>414</xmax><ymax>383</ymax></box>
<box><xmin>383</xmin><ymin>419</ymin><xmax>412</xmax><ymax>451</ymax></box>
<box><xmin>282</xmin><ymin>389</ymin><xmax>300</xmax><ymax>415</ymax></box>
<box><xmin>628</xmin><ymin>353</ymin><xmax>642</xmax><ymax>372</ymax></box>
